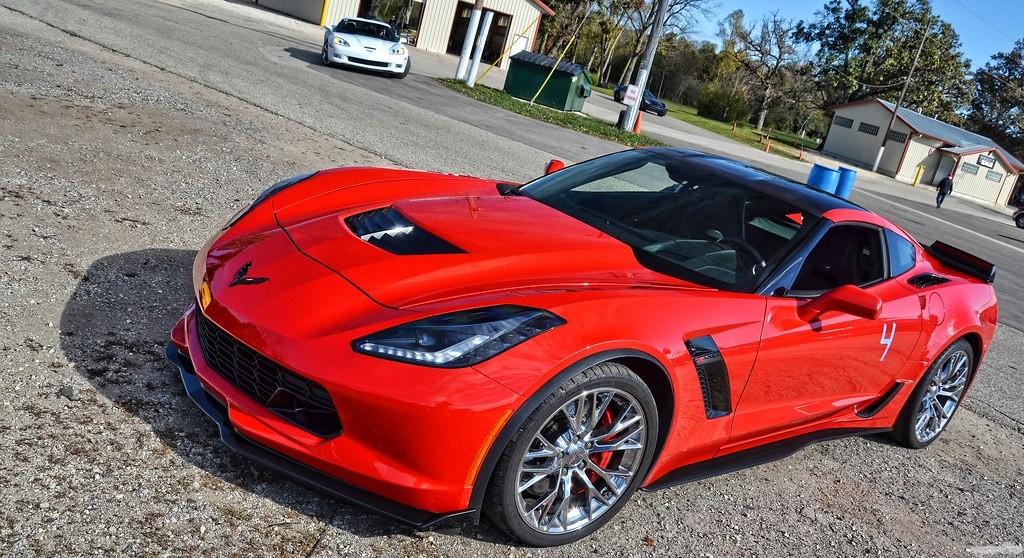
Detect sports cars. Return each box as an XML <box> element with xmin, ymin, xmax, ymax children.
<box><xmin>163</xmin><ymin>145</ymin><xmax>999</xmax><ymax>551</ymax></box>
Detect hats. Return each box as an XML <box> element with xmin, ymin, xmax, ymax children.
<box><xmin>948</xmin><ymin>174</ymin><xmax>953</xmax><ymax>176</ymax></box>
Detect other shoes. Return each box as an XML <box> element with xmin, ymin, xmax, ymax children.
<box><xmin>937</xmin><ymin>204</ymin><xmax>940</xmax><ymax>209</ymax></box>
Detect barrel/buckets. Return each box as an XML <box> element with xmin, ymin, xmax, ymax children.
<box><xmin>806</xmin><ymin>163</ymin><xmax>842</xmax><ymax>195</ymax></box>
<box><xmin>835</xmin><ymin>166</ymin><xmax>857</xmax><ymax>200</ymax></box>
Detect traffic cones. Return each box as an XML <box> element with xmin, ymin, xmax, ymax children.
<box><xmin>757</xmin><ymin>133</ymin><xmax>765</xmax><ymax>144</ymax></box>
<box><xmin>764</xmin><ymin>141</ymin><xmax>772</xmax><ymax>153</ymax></box>
<box><xmin>731</xmin><ymin>122</ymin><xmax>738</xmax><ymax>133</ymax></box>
<box><xmin>793</xmin><ymin>137</ymin><xmax>799</xmax><ymax>147</ymax></box>
<box><xmin>633</xmin><ymin>110</ymin><xmax>643</xmax><ymax>134</ymax></box>
<box><xmin>797</xmin><ymin>147</ymin><xmax>806</xmax><ymax>160</ymax></box>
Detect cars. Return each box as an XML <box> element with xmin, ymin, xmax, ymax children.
<box><xmin>1012</xmin><ymin>207</ymin><xmax>1024</xmax><ymax>229</ymax></box>
<box><xmin>323</xmin><ymin>17</ymin><xmax>411</xmax><ymax>79</ymax></box>
<box><xmin>614</xmin><ymin>84</ymin><xmax>668</xmax><ymax>117</ymax></box>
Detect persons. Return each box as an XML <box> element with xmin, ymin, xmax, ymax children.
<box><xmin>377</xmin><ymin>28</ymin><xmax>384</xmax><ymax>36</ymax></box>
<box><xmin>936</xmin><ymin>174</ymin><xmax>953</xmax><ymax>209</ymax></box>
